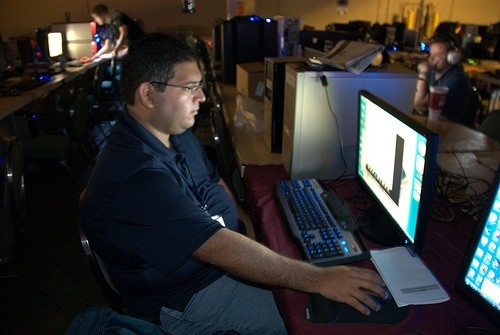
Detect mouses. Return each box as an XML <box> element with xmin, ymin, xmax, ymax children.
<box><xmin>39</xmin><ymin>75</ymin><xmax>53</xmax><ymax>81</ymax></box>
<box><xmin>359</xmin><ymin>284</ymin><xmax>393</xmax><ymax>304</ymax></box>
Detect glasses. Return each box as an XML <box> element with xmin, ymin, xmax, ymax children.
<box><xmin>150</xmin><ymin>81</ymin><xmax>203</xmax><ymax>98</ymax></box>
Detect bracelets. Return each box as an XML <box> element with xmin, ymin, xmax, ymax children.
<box><xmin>419</xmin><ymin>71</ymin><xmax>428</xmax><ymax>76</ymax></box>
<box><xmin>418</xmin><ymin>77</ymin><xmax>428</xmax><ymax>84</ymax></box>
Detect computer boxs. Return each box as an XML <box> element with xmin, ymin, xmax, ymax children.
<box><xmin>218</xmin><ymin>16</ymin><xmax>277</xmax><ymax>84</ymax></box>
<box><xmin>274</xmin><ymin>15</ymin><xmax>301</xmax><ymax>57</ymax></box>
<box><xmin>50</xmin><ymin>22</ymin><xmax>94</xmax><ymax>61</ymax></box>
<box><xmin>261</xmin><ymin>56</ymin><xmax>418</xmax><ymax>179</ymax></box>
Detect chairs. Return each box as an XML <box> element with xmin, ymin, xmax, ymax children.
<box><xmin>0</xmin><ymin>48</ymin><xmax>137</xmax><ymax>318</ymax></box>
<box><xmin>200</xmin><ymin>58</ymin><xmax>246</xmax><ymax>205</ymax></box>
<box><xmin>472</xmin><ymin>84</ymin><xmax>497</xmax><ymax>127</ymax></box>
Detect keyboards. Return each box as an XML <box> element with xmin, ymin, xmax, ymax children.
<box><xmin>12</xmin><ymin>79</ymin><xmax>46</xmax><ymax>90</ymax></box>
<box><xmin>278</xmin><ymin>177</ymin><xmax>372</xmax><ymax>267</ymax></box>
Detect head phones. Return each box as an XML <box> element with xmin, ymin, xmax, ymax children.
<box><xmin>433</xmin><ymin>35</ymin><xmax>462</xmax><ymax>65</ymax></box>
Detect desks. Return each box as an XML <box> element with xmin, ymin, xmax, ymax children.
<box><xmin>0</xmin><ymin>58</ymin><xmax>105</xmax><ymax>178</ymax></box>
<box><xmin>217</xmin><ymin>82</ymin><xmax>500</xmax><ymax>335</ymax></box>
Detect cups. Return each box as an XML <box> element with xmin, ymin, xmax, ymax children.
<box><xmin>428</xmin><ymin>86</ymin><xmax>449</xmax><ymax>122</ymax></box>
<box><xmin>64</xmin><ymin>11</ymin><xmax>71</xmax><ymax>23</ymax></box>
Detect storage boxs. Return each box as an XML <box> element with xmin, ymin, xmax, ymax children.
<box><xmin>237</xmin><ymin>62</ymin><xmax>266</xmax><ymax>96</ymax></box>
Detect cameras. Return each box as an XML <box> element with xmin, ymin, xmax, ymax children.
<box><xmin>354</xmin><ymin>87</ymin><xmax>439</xmax><ymax>256</ymax></box>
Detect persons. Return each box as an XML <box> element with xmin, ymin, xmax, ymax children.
<box><xmin>79</xmin><ymin>36</ymin><xmax>388</xmax><ymax>335</ymax></box>
<box><xmin>91</xmin><ymin>5</ymin><xmax>146</xmax><ymax>59</ymax></box>
<box><xmin>414</xmin><ymin>38</ymin><xmax>475</xmax><ymax>125</ymax></box>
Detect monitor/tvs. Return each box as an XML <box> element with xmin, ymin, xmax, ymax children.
<box><xmin>46</xmin><ymin>31</ymin><xmax>63</xmax><ymax>58</ymax></box>
